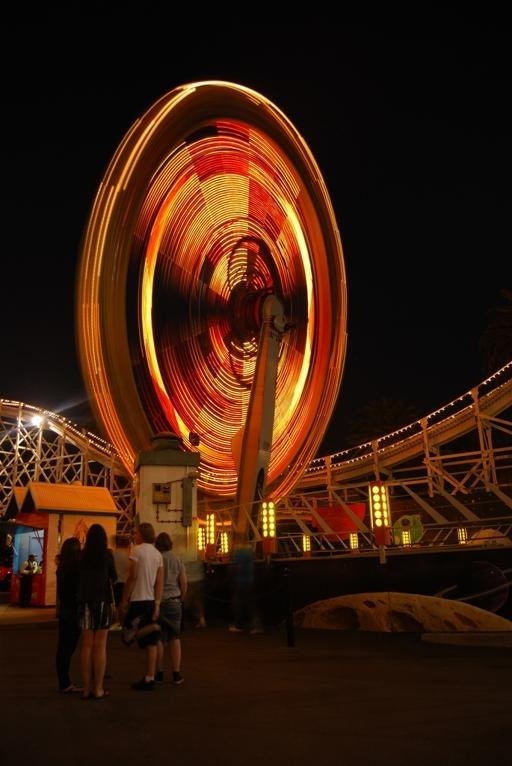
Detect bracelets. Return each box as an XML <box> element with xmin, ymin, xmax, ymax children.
<box><xmin>154</xmin><ymin>599</ymin><xmax>162</xmax><ymax>605</ymax></box>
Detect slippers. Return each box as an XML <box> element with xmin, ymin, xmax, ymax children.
<box><xmin>62</xmin><ymin>687</ymin><xmax>109</xmax><ymax>700</ymax></box>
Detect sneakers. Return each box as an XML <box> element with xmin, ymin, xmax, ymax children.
<box><xmin>154</xmin><ymin>615</ymin><xmax>179</xmax><ymax>634</ymax></box>
<box><xmin>131</xmin><ymin>670</ymin><xmax>186</xmax><ymax>691</ymax></box>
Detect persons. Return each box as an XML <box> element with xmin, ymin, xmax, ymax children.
<box><xmin>55</xmin><ymin>536</ymin><xmax>84</xmax><ymax>694</ymax></box>
<box><xmin>153</xmin><ymin>531</ymin><xmax>189</xmax><ymax>686</ymax></box>
<box><xmin>80</xmin><ymin>524</ymin><xmax>118</xmax><ymax>699</ymax></box>
<box><xmin>174</xmin><ymin>534</ymin><xmax>209</xmax><ymax>631</ymax></box>
<box><xmin>109</xmin><ymin>532</ymin><xmax>130</xmax><ymax>632</ymax></box>
<box><xmin>121</xmin><ymin>522</ymin><xmax>164</xmax><ymax>691</ymax></box>
<box><xmin>18</xmin><ymin>553</ymin><xmax>38</xmax><ymax>607</ymax></box>
<box><xmin>225</xmin><ymin>531</ymin><xmax>265</xmax><ymax>636</ymax></box>
